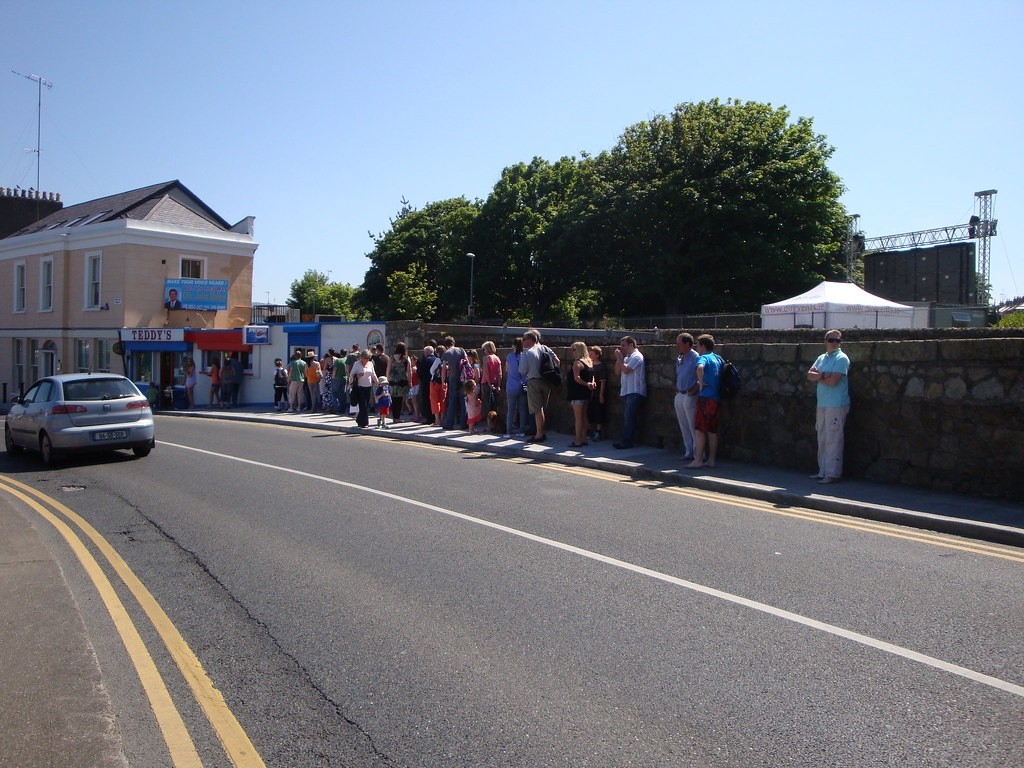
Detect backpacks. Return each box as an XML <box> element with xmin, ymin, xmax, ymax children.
<box><xmin>275</xmin><ymin>368</ymin><xmax>287</xmax><ymax>386</ymax></box>
<box><xmin>433</xmin><ymin>357</ymin><xmax>449</xmax><ymax>383</ymax></box>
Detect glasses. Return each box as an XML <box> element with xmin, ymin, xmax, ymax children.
<box><xmin>828</xmin><ymin>338</ymin><xmax>841</xmax><ymax>344</ymax></box>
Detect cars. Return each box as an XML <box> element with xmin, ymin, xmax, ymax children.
<box><xmin>4</xmin><ymin>372</ymin><xmax>156</xmax><ymax>467</ymax></box>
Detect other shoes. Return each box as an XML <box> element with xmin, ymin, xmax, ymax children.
<box><xmin>592</xmin><ymin>433</ymin><xmax>602</xmax><ymax>442</ymax></box>
<box><xmin>680</xmin><ymin>456</ymin><xmax>707</xmax><ymax>461</ymax></box>
<box><xmin>809</xmin><ymin>474</ymin><xmax>822</xmax><ymax>479</ymax></box>
<box><xmin>527</xmin><ymin>434</ymin><xmax>546</xmax><ymax>442</ymax></box>
<box><xmin>585</xmin><ymin>434</ymin><xmax>594</xmax><ymax>439</ymax></box>
<box><xmin>613</xmin><ymin>441</ymin><xmax>634</xmax><ymax>449</ymax></box>
<box><xmin>818</xmin><ymin>477</ymin><xmax>840</xmax><ymax>484</ymax></box>
<box><xmin>273</xmin><ymin>404</ymin><xmax>537</xmax><ymax>438</ymax></box>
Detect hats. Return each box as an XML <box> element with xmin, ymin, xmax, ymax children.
<box><xmin>379</xmin><ymin>376</ymin><xmax>389</xmax><ymax>384</ymax></box>
<box><xmin>306</xmin><ymin>351</ymin><xmax>317</xmax><ymax>358</ymax></box>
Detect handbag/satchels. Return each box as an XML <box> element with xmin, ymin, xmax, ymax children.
<box><xmin>350</xmin><ymin>374</ymin><xmax>360</xmax><ymax>406</ymax></box>
<box><xmin>719</xmin><ymin>355</ymin><xmax>740</xmax><ymax>399</ymax></box>
<box><xmin>539</xmin><ymin>345</ymin><xmax>561</xmax><ymax>386</ymax></box>
<box><xmin>521</xmin><ymin>382</ymin><xmax>528</xmax><ymax>393</ymax></box>
<box><xmin>459</xmin><ymin>347</ymin><xmax>476</xmax><ymax>385</ymax></box>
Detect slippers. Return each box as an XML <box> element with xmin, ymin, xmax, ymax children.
<box><xmin>702</xmin><ymin>463</ymin><xmax>716</xmax><ymax>469</ymax></box>
<box><xmin>568</xmin><ymin>440</ymin><xmax>588</xmax><ymax>448</ymax></box>
<box><xmin>682</xmin><ymin>464</ymin><xmax>703</xmax><ymax>469</ymax></box>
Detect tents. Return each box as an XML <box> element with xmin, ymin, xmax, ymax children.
<box><xmin>761</xmin><ymin>280</ymin><xmax>916</xmax><ymax>332</ymax></box>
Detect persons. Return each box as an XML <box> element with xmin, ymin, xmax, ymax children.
<box><xmin>390</xmin><ymin>335</ymin><xmax>502</xmax><ymax>435</ymax></box>
<box><xmin>165</xmin><ymin>288</ymin><xmax>181</xmax><ymax>308</ymax></box>
<box><xmin>807</xmin><ymin>328</ymin><xmax>851</xmax><ymax>484</ymax></box>
<box><xmin>185</xmin><ymin>358</ymin><xmax>197</xmax><ymax>410</ymax></box>
<box><xmin>504</xmin><ymin>337</ymin><xmax>528</xmax><ymax>438</ymax></box>
<box><xmin>218</xmin><ymin>351</ymin><xmax>242</xmax><ymax>409</ymax></box>
<box><xmin>684</xmin><ymin>334</ymin><xmax>726</xmax><ymax>470</ymax></box>
<box><xmin>517</xmin><ymin>330</ymin><xmax>560</xmax><ymax>444</ymax></box>
<box><xmin>205</xmin><ymin>358</ymin><xmax>223</xmax><ymax>409</ymax></box>
<box><xmin>143</xmin><ymin>381</ymin><xmax>160</xmax><ymax>405</ymax></box>
<box><xmin>674</xmin><ymin>332</ymin><xmax>707</xmax><ymax>461</ymax></box>
<box><xmin>566</xmin><ymin>342</ymin><xmax>597</xmax><ymax>446</ymax></box>
<box><xmin>272</xmin><ymin>345</ymin><xmax>391</xmax><ymax>429</ymax></box>
<box><xmin>613</xmin><ymin>336</ymin><xmax>646</xmax><ymax>449</ymax></box>
<box><xmin>585</xmin><ymin>346</ymin><xmax>608</xmax><ymax>442</ymax></box>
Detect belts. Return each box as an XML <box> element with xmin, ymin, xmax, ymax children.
<box><xmin>680</xmin><ymin>391</ymin><xmax>687</xmax><ymax>394</ymax></box>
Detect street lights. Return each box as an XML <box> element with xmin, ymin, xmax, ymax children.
<box><xmin>466</xmin><ymin>253</ymin><xmax>476</xmax><ymax>321</ymax></box>
